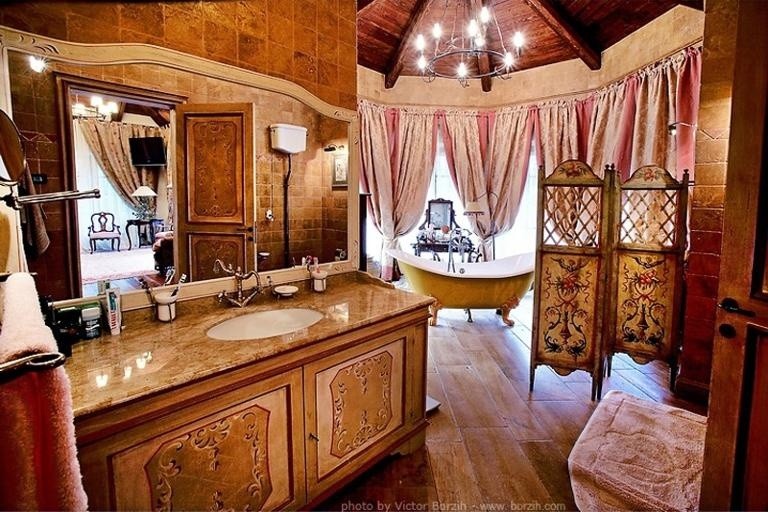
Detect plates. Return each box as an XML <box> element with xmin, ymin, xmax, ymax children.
<box><xmin>274</xmin><ymin>285</ymin><xmax>298</xmax><ymax>297</ymax></box>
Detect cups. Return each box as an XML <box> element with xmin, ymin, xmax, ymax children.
<box><xmin>153</xmin><ymin>292</ymin><xmax>177</xmax><ymax>322</ymax></box>
<box><xmin>313</xmin><ymin>270</ymin><xmax>327</xmax><ymax>291</ymax></box>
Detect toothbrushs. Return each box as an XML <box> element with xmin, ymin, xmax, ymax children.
<box><xmin>165</xmin><ymin>269</ymin><xmax>175</xmax><ymax>285</ymax></box>
<box><xmin>314</xmin><ymin>257</ymin><xmax>318</xmax><ymax>270</ymax></box>
<box><xmin>171</xmin><ymin>274</ymin><xmax>187</xmax><ymax>296</ymax></box>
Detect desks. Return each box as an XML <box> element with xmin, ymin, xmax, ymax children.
<box><xmin>125</xmin><ymin>220</ymin><xmax>164</xmax><ymax>250</ymax></box>
<box><xmin>411</xmin><ymin>240</ymin><xmax>474</xmax><ymax>263</ymax></box>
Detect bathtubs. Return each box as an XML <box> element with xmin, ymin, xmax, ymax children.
<box><xmin>384</xmin><ymin>249</ymin><xmax>538</xmax><ymax>326</ymax></box>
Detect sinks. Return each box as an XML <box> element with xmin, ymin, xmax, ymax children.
<box><xmin>205</xmin><ymin>306</ymin><xmax>325</xmax><ymax>341</ymax></box>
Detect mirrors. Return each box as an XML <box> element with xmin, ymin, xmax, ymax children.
<box><xmin>427</xmin><ymin>198</ymin><xmax>454</xmax><ymax>232</ymax></box>
<box><xmin>1</xmin><ymin>27</ymin><xmax>360</xmax><ymax>317</ymax></box>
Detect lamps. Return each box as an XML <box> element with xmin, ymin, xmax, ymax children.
<box><xmin>412</xmin><ymin>0</ymin><xmax>527</xmax><ymax>86</ymax></box>
<box><xmin>131</xmin><ymin>186</ymin><xmax>159</xmax><ymax>219</ymax></box>
<box><xmin>324</xmin><ymin>144</ymin><xmax>344</xmax><ymax>152</ymax></box>
<box><xmin>462</xmin><ymin>191</ymin><xmax>500</xmax><ymax>221</ymax></box>
<box><xmin>72</xmin><ymin>92</ymin><xmax>120</xmax><ymax>123</ymax></box>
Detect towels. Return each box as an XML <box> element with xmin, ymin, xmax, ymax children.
<box><xmin>2</xmin><ymin>272</ymin><xmax>89</xmax><ymax>510</ymax></box>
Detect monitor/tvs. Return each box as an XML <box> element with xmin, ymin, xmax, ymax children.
<box><xmin>128</xmin><ymin>137</ymin><xmax>166</xmax><ymax>167</ymax></box>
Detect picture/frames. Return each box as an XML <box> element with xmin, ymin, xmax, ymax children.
<box><xmin>332</xmin><ymin>151</ymin><xmax>347</xmax><ymax>190</ymax></box>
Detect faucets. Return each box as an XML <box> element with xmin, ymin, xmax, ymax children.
<box><xmin>235</xmin><ymin>266</ymin><xmax>266</xmax><ymax>302</ymax></box>
<box><xmin>212</xmin><ymin>259</ymin><xmax>236</xmax><ymax>276</ymax></box>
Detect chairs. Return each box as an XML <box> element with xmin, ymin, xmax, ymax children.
<box><xmin>88</xmin><ymin>211</ymin><xmax>122</xmax><ymax>254</ymax></box>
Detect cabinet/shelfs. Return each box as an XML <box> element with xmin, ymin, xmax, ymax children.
<box><xmin>75</xmin><ymin>307</ymin><xmax>434</xmax><ymax>512</ymax></box>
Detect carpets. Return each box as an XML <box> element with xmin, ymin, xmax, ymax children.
<box><xmin>80</xmin><ymin>246</ymin><xmax>159</xmax><ymax>284</ymax></box>
<box><xmin>569</xmin><ymin>389</ymin><xmax>706</xmax><ymax>511</ymax></box>
